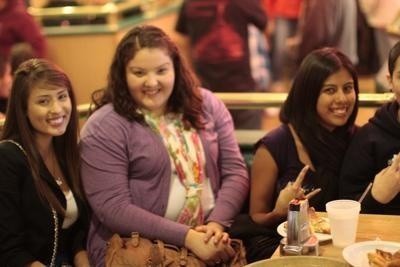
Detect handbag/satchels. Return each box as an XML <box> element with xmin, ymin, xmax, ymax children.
<box><xmin>104</xmin><ymin>232</ymin><xmax>247</xmax><ymax>267</ymax></box>
<box><xmin>247</xmin><ymin>24</ymin><xmax>272</xmax><ymax>87</ymax></box>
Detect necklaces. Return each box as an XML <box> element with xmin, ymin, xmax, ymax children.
<box><xmin>51</xmin><ymin>148</ymin><xmax>65</xmax><ymax>186</ymax></box>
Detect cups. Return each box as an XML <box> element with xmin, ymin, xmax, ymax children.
<box><xmin>326</xmin><ymin>200</ymin><xmax>361</xmax><ymax>247</ymax></box>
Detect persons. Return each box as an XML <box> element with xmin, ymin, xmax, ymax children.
<box><xmin>0</xmin><ymin>59</ymin><xmax>93</xmax><ymax>267</ymax></box>
<box><xmin>244</xmin><ymin>47</ymin><xmax>361</xmax><ymax>262</ymax></box>
<box><xmin>77</xmin><ymin>26</ymin><xmax>250</xmax><ymax>267</ymax></box>
<box><xmin>174</xmin><ymin>0</ymin><xmax>269</xmax><ymax>131</ymax></box>
<box><xmin>0</xmin><ymin>1</ymin><xmax>47</xmax><ymax>59</ymax></box>
<box><xmin>0</xmin><ymin>53</ymin><xmax>13</xmax><ymax>134</ymax></box>
<box><xmin>296</xmin><ymin>0</ymin><xmax>360</xmax><ymax>69</ymax></box>
<box><xmin>338</xmin><ymin>41</ymin><xmax>400</xmax><ymax>215</ymax></box>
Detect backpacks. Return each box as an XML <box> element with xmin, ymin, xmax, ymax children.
<box><xmin>193</xmin><ymin>0</ymin><xmax>245</xmax><ymax>80</ymax></box>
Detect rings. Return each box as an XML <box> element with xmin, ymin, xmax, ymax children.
<box><xmin>214</xmin><ymin>257</ymin><xmax>223</xmax><ymax>264</ymax></box>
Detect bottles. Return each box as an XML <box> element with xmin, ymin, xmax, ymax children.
<box><xmin>283</xmin><ymin>204</ymin><xmax>303</xmax><ymax>257</ymax></box>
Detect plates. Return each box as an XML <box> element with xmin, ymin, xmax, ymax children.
<box><xmin>277</xmin><ymin>221</ymin><xmax>332</xmax><ymax>242</ymax></box>
<box><xmin>342</xmin><ymin>240</ymin><xmax>399</xmax><ymax>267</ymax></box>
